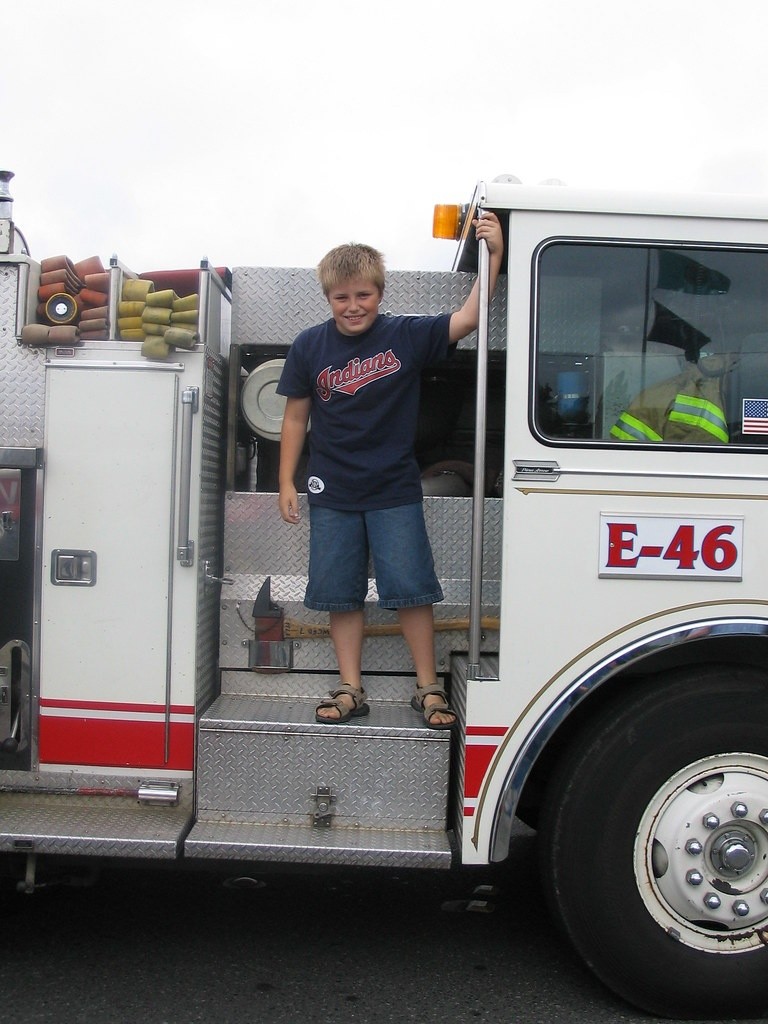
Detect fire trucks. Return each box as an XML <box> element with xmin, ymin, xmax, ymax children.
<box><xmin>1</xmin><ymin>169</ymin><xmax>768</xmax><ymax>1024</ymax></box>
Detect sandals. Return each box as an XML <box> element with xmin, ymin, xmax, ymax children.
<box><xmin>315</xmin><ymin>687</ymin><xmax>371</xmax><ymax>725</ymax></box>
<box><xmin>411</xmin><ymin>681</ymin><xmax>459</xmax><ymax>730</ymax></box>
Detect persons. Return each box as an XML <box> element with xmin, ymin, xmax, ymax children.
<box><xmin>608</xmin><ymin>323</ymin><xmax>729</xmax><ymax>444</ymax></box>
<box><xmin>275</xmin><ymin>212</ymin><xmax>504</xmax><ymax>729</ymax></box>
